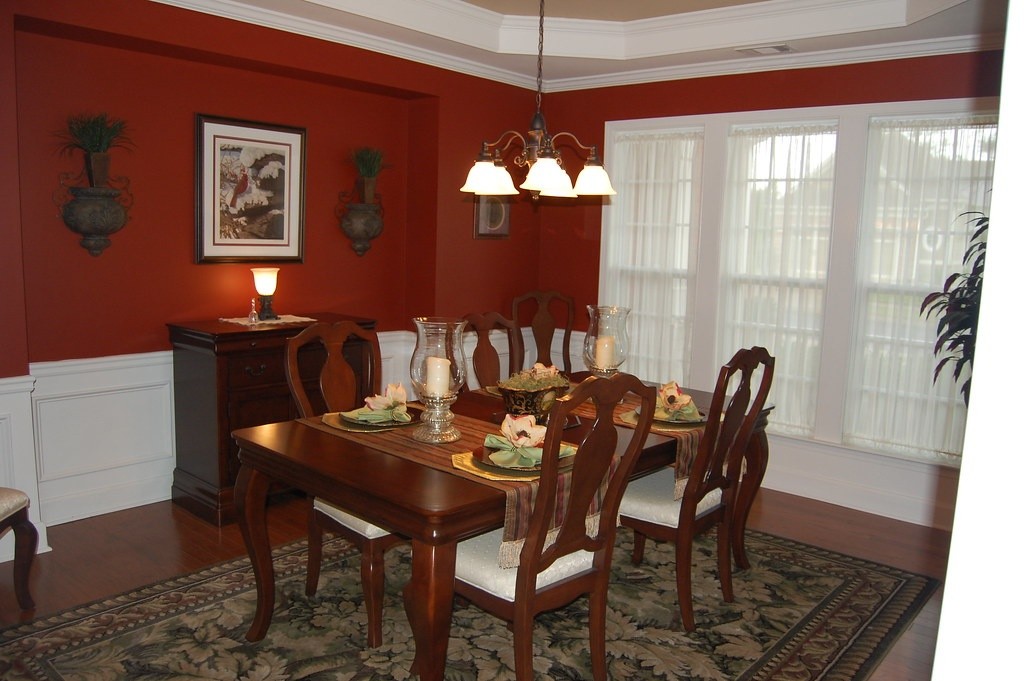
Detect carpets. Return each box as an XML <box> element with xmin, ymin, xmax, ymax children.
<box><xmin>0</xmin><ymin>524</ymin><xmax>942</xmax><ymax>681</ymax></box>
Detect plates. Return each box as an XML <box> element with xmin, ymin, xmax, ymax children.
<box><xmin>339</xmin><ymin>406</ymin><xmax>423</xmax><ymax>426</ymax></box>
<box><xmin>472</xmin><ymin>446</ymin><xmax>574</xmax><ymax>471</ymax></box>
<box><xmin>635</xmin><ymin>404</ymin><xmax>710</xmax><ymax>423</ymax></box>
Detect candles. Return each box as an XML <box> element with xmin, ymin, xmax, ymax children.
<box><xmin>427</xmin><ymin>356</ymin><xmax>451</xmax><ymax>397</ymax></box>
<box><xmin>596</xmin><ymin>337</ymin><xmax>615</xmax><ymax>369</ymax></box>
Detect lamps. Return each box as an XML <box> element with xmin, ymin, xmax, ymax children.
<box><xmin>460</xmin><ymin>0</ymin><xmax>617</xmax><ymax>201</ymax></box>
<box><xmin>251</xmin><ymin>268</ymin><xmax>281</xmax><ymax>321</ymax></box>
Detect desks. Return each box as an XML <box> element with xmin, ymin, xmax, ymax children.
<box><xmin>230</xmin><ymin>371</ymin><xmax>775</xmax><ymax>681</ymax></box>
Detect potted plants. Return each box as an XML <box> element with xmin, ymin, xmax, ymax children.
<box><xmin>51</xmin><ymin>112</ymin><xmax>138</xmax><ymax>188</ymax></box>
<box><xmin>346</xmin><ymin>147</ymin><xmax>390</xmax><ymax>205</ymax></box>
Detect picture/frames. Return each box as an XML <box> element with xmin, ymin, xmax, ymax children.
<box><xmin>473</xmin><ymin>195</ymin><xmax>511</xmax><ymax>240</ymax></box>
<box><xmin>195</xmin><ymin>112</ymin><xmax>307</xmax><ymax>264</ymax></box>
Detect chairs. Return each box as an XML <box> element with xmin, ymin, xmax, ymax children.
<box><xmin>285</xmin><ymin>320</ymin><xmax>411</xmax><ymax>648</ymax></box>
<box><xmin>619</xmin><ymin>346</ymin><xmax>775</xmax><ymax>631</ymax></box>
<box><xmin>404</xmin><ymin>373</ymin><xmax>656</xmax><ymax>681</ymax></box>
<box><xmin>1</xmin><ymin>488</ymin><xmax>39</xmax><ymax>611</ymax></box>
<box><xmin>445</xmin><ymin>290</ymin><xmax>575</xmax><ymax>393</ymax></box>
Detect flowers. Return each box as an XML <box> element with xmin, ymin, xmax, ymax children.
<box><xmin>364</xmin><ymin>382</ymin><xmax>406</xmax><ymax>412</ymax></box>
<box><xmin>656</xmin><ymin>382</ymin><xmax>692</xmax><ymax>411</ymax></box>
<box><xmin>499</xmin><ymin>413</ymin><xmax>547</xmax><ymax>448</ymax></box>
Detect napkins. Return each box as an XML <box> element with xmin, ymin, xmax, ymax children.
<box><xmin>341</xmin><ymin>405</ymin><xmax>411</xmax><ymax>423</ymax></box>
<box><xmin>484</xmin><ymin>434</ymin><xmax>578</xmax><ymax>468</ymax></box>
<box><xmin>654</xmin><ymin>401</ymin><xmax>700</xmax><ymax>421</ymax></box>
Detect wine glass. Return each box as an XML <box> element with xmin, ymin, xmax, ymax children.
<box><xmin>249</xmin><ymin>298</ymin><xmax>258</xmax><ymax>323</ymax></box>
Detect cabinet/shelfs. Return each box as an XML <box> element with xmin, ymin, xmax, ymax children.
<box><xmin>165</xmin><ymin>313</ymin><xmax>376</xmax><ymax>527</ymax></box>
